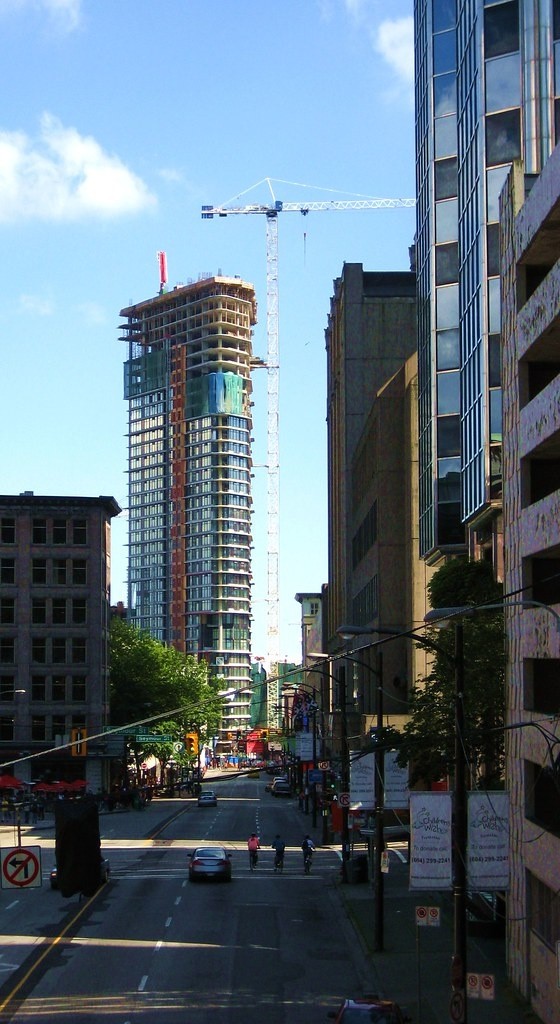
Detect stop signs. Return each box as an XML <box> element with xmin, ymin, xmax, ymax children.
<box><xmin>142</xmin><ymin>763</ymin><xmax>147</xmax><ymax>768</ymax></box>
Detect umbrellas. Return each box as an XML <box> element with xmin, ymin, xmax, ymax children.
<box><xmin>0</xmin><ymin>773</ymin><xmax>89</xmax><ymax>792</ymax></box>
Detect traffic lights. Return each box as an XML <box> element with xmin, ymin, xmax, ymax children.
<box><xmin>186</xmin><ymin>733</ymin><xmax>198</xmax><ymax>755</ymax></box>
<box><xmin>228</xmin><ymin>732</ymin><xmax>232</xmax><ymax>739</ymax></box>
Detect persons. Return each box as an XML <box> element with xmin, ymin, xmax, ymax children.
<box><xmin>1</xmin><ymin>758</ymin><xmax>333</xmax><ymax>823</ymax></box>
<box><xmin>248</xmin><ymin>833</ymin><xmax>260</xmax><ymax>869</ymax></box>
<box><xmin>301</xmin><ymin>835</ymin><xmax>315</xmax><ymax>872</ymax></box>
<box><xmin>271</xmin><ymin>835</ymin><xmax>285</xmax><ymax>872</ymax></box>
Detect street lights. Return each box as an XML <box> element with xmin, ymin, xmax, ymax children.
<box><xmin>306</xmin><ymin>652</ymin><xmax>385</xmax><ymax>953</ymax></box>
<box><xmin>279</xmin><ymin>695</ymin><xmax>303</xmax><ymax>701</ymax></box>
<box><xmin>337</xmin><ymin>624</ymin><xmax>465</xmax><ymax>1024</ymax></box>
<box><xmin>283</xmin><ymin>682</ymin><xmax>328</xmax><ymax>846</ymax></box>
<box><xmin>280</xmin><ymin>688</ymin><xmax>318</xmax><ymax>828</ymax></box>
<box><xmin>287</xmin><ymin>668</ymin><xmax>350</xmax><ymax>870</ymax></box>
<box><xmin>285</xmin><ymin>663</ymin><xmax>350</xmax><ymax>862</ymax></box>
<box><xmin>424</xmin><ymin>601</ymin><xmax>560</xmax><ymax>628</ymax></box>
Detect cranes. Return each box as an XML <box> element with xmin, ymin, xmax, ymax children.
<box><xmin>201</xmin><ymin>177</ymin><xmax>416</xmax><ymax>663</ymax></box>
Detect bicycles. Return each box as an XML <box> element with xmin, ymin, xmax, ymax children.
<box><xmin>274</xmin><ymin>847</ymin><xmax>283</xmax><ymax>873</ymax></box>
<box><xmin>304</xmin><ymin>851</ymin><xmax>311</xmax><ymax>872</ymax></box>
<box><xmin>249</xmin><ymin>848</ymin><xmax>260</xmax><ymax>869</ymax></box>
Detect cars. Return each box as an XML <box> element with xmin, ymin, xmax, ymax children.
<box><xmin>271</xmin><ymin>780</ymin><xmax>291</xmax><ymax>797</ymax></box>
<box><xmin>186</xmin><ymin>846</ymin><xmax>232</xmax><ymax>884</ymax></box>
<box><xmin>198</xmin><ymin>791</ymin><xmax>218</xmax><ymax>808</ymax></box>
<box><xmin>274</xmin><ymin>777</ymin><xmax>285</xmax><ymax>784</ymax></box>
<box><xmin>247</xmin><ymin>769</ymin><xmax>258</xmax><ymax>777</ymax></box>
